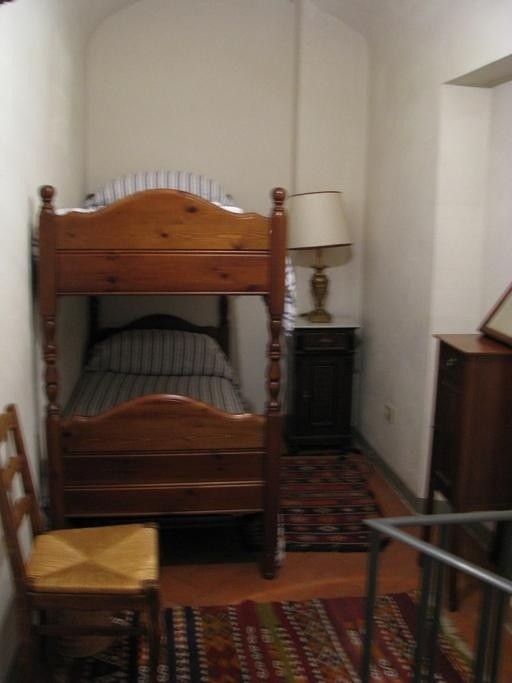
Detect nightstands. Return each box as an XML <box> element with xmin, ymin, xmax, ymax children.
<box><xmin>291</xmin><ymin>313</ymin><xmax>364</xmax><ymax>456</ymax></box>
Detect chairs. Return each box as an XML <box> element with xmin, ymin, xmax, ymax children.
<box><xmin>0</xmin><ymin>400</ymin><xmax>163</xmax><ymax>682</ymax></box>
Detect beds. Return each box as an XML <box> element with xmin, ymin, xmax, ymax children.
<box><xmin>35</xmin><ymin>185</ymin><xmax>291</xmax><ymax>581</ymax></box>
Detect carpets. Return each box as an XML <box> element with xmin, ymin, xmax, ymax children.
<box><xmin>278</xmin><ymin>448</ymin><xmax>391</xmax><ymax>554</ymax></box>
<box><xmin>32</xmin><ymin>592</ymin><xmax>487</xmax><ymax>683</ymax></box>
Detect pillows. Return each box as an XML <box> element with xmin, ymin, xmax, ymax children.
<box><xmin>83</xmin><ymin>168</ymin><xmax>235</xmax><ymax>207</ymax></box>
<box><xmin>87</xmin><ymin>326</ymin><xmax>238</xmax><ymax>378</ymax></box>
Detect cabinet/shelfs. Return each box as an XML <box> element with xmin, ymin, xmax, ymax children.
<box><xmin>415</xmin><ymin>332</ymin><xmax>512</xmax><ymax>611</ymax></box>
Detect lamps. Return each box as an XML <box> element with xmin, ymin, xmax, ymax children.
<box><xmin>288</xmin><ymin>187</ymin><xmax>355</xmax><ymax>322</ymax></box>
